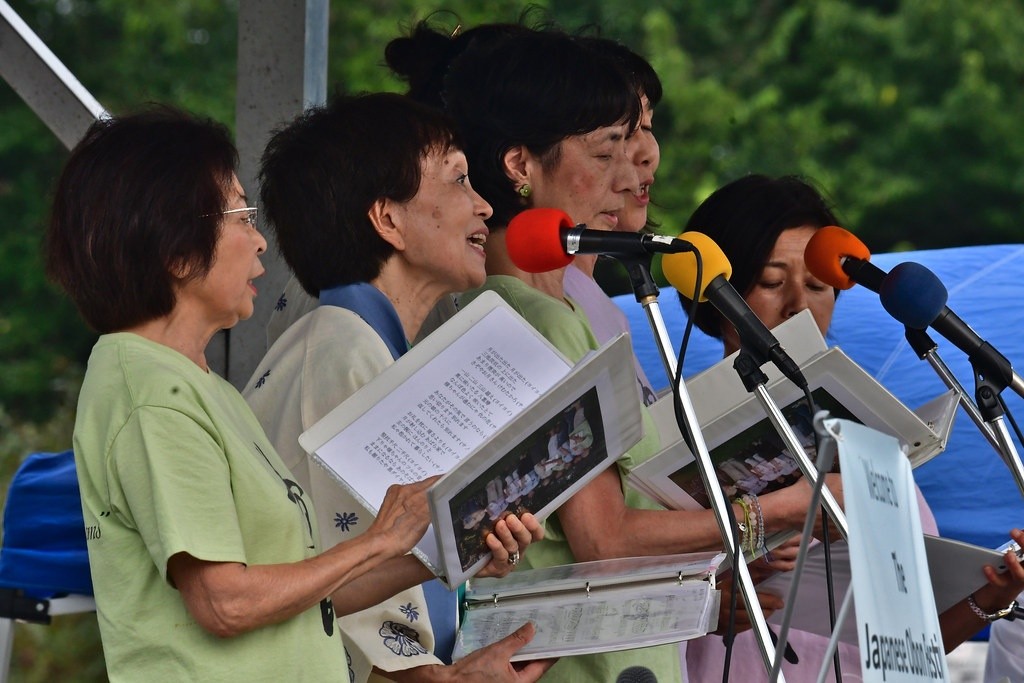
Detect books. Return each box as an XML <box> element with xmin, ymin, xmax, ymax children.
<box><xmin>755</xmin><ymin>533</ymin><xmax>1024</xmax><ymax>646</ymax></box>
<box><xmin>451</xmin><ymin>551</ymin><xmax>728</xmax><ymax>665</ymax></box>
<box><xmin>623</xmin><ymin>307</ymin><xmax>963</xmax><ymax>567</ymax></box>
<box><xmin>298</xmin><ymin>290</ymin><xmax>648</xmax><ymax>592</ymax></box>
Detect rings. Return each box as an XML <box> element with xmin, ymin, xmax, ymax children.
<box><xmin>508</xmin><ymin>549</ymin><xmax>521</xmax><ymax>567</ymax></box>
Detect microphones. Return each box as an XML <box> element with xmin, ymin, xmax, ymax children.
<box><xmin>662</xmin><ymin>231</ymin><xmax>809</xmax><ymax>388</ymax></box>
<box><xmin>804</xmin><ymin>225</ymin><xmax>888</xmax><ymax>294</ymax></box>
<box><xmin>880</xmin><ymin>261</ymin><xmax>1024</xmax><ymax>399</ymax></box>
<box><xmin>505</xmin><ymin>208</ymin><xmax>694</xmax><ymax>273</ymax></box>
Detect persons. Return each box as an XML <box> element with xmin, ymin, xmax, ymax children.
<box><xmin>676</xmin><ymin>174</ymin><xmax>1024</xmax><ymax>683</ymax></box>
<box><xmin>580</xmin><ymin>38</ymin><xmax>814</xmax><ymax>683</ymax></box>
<box><xmin>690</xmin><ymin>399</ymin><xmax>832</xmax><ymax>503</ymax></box>
<box><xmin>456</xmin><ymin>400</ymin><xmax>594</xmax><ymax>558</ymax></box>
<box><xmin>38</xmin><ymin>101</ymin><xmax>547</xmax><ymax>683</ymax></box>
<box><xmin>240</xmin><ymin>91</ymin><xmax>561</xmax><ymax>683</ymax></box>
<box><xmin>378</xmin><ymin>8</ymin><xmax>844</xmax><ymax>683</ymax></box>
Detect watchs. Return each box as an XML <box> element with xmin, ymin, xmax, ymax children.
<box><xmin>967</xmin><ymin>593</ymin><xmax>1015</xmax><ymax>622</ymax></box>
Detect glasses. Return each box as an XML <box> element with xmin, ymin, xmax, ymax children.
<box><xmin>196</xmin><ymin>200</ymin><xmax>259</xmax><ymax>230</ymax></box>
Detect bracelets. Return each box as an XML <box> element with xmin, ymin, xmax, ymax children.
<box><xmin>733</xmin><ymin>491</ymin><xmax>764</xmax><ymax>553</ymax></box>
